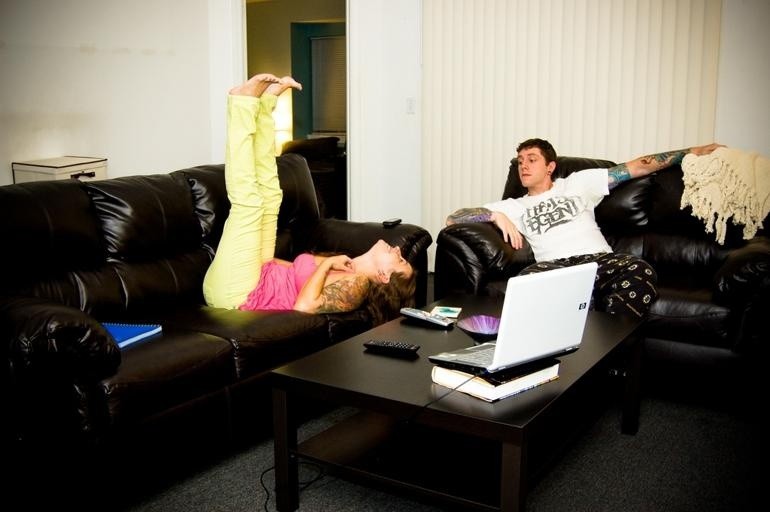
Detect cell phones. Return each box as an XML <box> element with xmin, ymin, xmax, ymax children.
<box><xmin>382</xmin><ymin>217</ymin><xmax>402</xmax><ymax>227</ymax></box>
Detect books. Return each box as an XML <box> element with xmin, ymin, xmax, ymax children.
<box><xmin>431</xmin><ymin>359</ymin><xmax>561</xmax><ymax>403</ymax></box>
<box><xmin>102</xmin><ymin>322</ymin><xmax>162</xmax><ymax>348</ymax></box>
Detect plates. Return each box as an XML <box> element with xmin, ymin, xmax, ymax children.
<box><xmin>454</xmin><ymin>314</ymin><xmax>502</xmax><ymax>346</ymax></box>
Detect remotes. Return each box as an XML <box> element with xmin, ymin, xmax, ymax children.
<box><xmin>400</xmin><ymin>308</ymin><xmax>454</xmax><ymax>328</ymax></box>
<box><xmin>363</xmin><ymin>339</ymin><xmax>421</xmax><ymax>352</ymax></box>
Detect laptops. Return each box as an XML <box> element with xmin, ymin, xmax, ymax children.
<box><xmin>427</xmin><ymin>262</ymin><xmax>598</xmax><ymax>377</ymax></box>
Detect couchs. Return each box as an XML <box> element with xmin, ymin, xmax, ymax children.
<box><xmin>0</xmin><ymin>154</ymin><xmax>432</xmax><ymax>512</ymax></box>
<box><xmin>434</xmin><ymin>158</ymin><xmax>770</xmax><ymax>415</ymax></box>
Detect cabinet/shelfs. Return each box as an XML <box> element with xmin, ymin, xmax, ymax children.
<box><xmin>12</xmin><ymin>156</ymin><xmax>108</xmax><ymax>184</ymax></box>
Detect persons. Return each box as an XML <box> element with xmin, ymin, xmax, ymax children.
<box><xmin>202</xmin><ymin>73</ymin><xmax>417</xmax><ymax>327</ymax></box>
<box><xmin>445</xmin><ymin>138</ymin><xmax>727</xmax><ymax>321</ymax></box>
